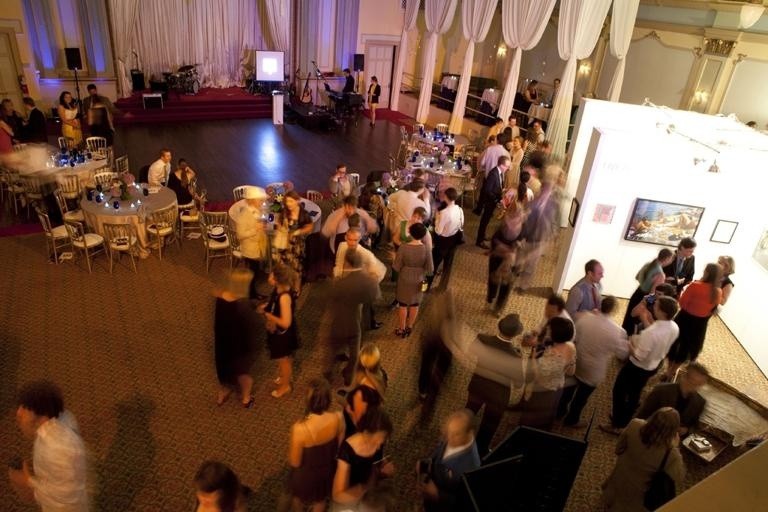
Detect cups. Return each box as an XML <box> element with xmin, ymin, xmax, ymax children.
<box><xmin>540</xmin><ymin>100</ymin><xmax>550</xmax><ymax>108</ymax></box>
<box><xmin>10</xmin><ymin>457</ymin><xmax>23</xmax><ymax>472</ymax></box>
<box><xmin>523</xmin><ymin>332</ymin><xmax>533</xmax><ymax>342</ymax></box>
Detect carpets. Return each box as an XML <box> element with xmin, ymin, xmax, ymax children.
<box><xmin>1</xmin><ymin>219</ymin><xmax>768</xmax><ymax>512</ymax></box>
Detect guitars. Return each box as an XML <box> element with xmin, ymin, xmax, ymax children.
<box><xmin>300</xmin><ymin>72</ymin><xmax>313</xmax><ymax>104</ymax></box>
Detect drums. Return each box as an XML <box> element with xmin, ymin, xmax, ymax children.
<box><xmin>186</xmin><ymin>80</ymin><xmax>198</xmax><ymax>94</ymax></box>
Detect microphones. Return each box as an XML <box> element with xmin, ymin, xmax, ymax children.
<box><xmin>310</xmin><ymin>59</ymin><xmax>317</xmax><ymax>67</ymax></box>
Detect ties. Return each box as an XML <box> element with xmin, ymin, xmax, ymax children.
<box><xmin>164</xmin><ymin>166</ymin><xmax>169</xmax><ymax>188</ymax></box>
<box><xmin>592</xmin><ymin>287</ymin><xmax>598</xmax><ymax>307</ymax></box>
<box><xmin>675</xmin><ymin>258</ymin><xmax>684</xmax><ymax>279</ymax></box>
<box><xmin>501</xmin><ymin>173</ymin><xmax>504</xmax><ymax>190</ymax></box>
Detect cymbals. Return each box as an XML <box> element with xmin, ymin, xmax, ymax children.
<box><xmin>178</xmin><ymin>65</ymin><xmax>193</xmax><ymax>72</ymax></box>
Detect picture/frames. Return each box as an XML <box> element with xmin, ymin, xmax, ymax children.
<box><xmin>568</xmin><ymin>197</ymin><xmax>579</xmax><ymax>227</ymax></box>
<box><xmin>710</xmin><ymin>220</ymin><xmax>740</xmax><ymax>245</ymax></box>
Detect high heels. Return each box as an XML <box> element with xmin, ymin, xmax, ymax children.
<box><xmin>273</xmin><ymin>375</ymin><xmax>294</xmax><ymax>385</ymax></box>
<box><xmin>395</xmin><ymin>326</ymin><xmax>406</xmax><ymax>338</ymax></box>
<box><xmin>405</xmin><ymin>327</ymin><xmax>412</xmax><ymax>337</ymax></box>
<box><xmin>271</xmin><ymin>384</ymin><xmax>292</xmax><ymax>398</ymax></box>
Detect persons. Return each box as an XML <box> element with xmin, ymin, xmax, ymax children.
<box><xmin>7</xmin><ymin>381</ymin><xmax>92</xmax><ymax>512</ymax></box>
<box><xmin>368</xmin><ymin>76</ymin><xmax>381</xmax><ymax>128</ymax></box>
<box><xmin>191</xmin><ymin>461</ymin><xmax>251</xmax><ymax>511</ymax></box>
<box><xmin>522</xmin><ymin>80</ymin><xmax>540</xmax><ymax>111</ymax></box>
<box><xmin>169</xmin><ymin>157</ymin><xmax>204</xmax><ymax>205</ymax></box>
<box><xmin>2</xmin><ymin>99</ymin><xmax>24</xmax><ymax>130</ymax></box>
<box><xmin>553</xmin><ymin>239</ymin><xmax>734</xmax><ymax>511</ymax></box>
<box><xmin>0</xmin><ymin>102</ymin><xmax>15</xmax><ymax>152</ymax></box>
<box><xmin>147</xmin><ymin>148</ymin><xmax>172</xmax><ymax>187</ymax></box>
<box><xmin>16</xmin><ymin>97</ymin><xmax>48</xmax><ymax>143</ymax></box>
<box><xmin>545</xmin><ymin>79</ymin><xmax>561</xmax><ymax>104</ymax></box>
<box><xmin>343</xmin><ymin>69</ymin><xmax>354</xmax><ymax>92</ymax></box>
<box><xmin>58</xmin><ymin>91</ymin><xmax>85</xmax><ymax>147</ymax></box>
<box><xmin>212</xmin><ymin>111</ymin><xmax>555</xmax><ymax>512</ymax></box>
<box><xmin>84</xmin><ymin>84</ymin><xmax>116</xmax><ymax>147</ymax></box>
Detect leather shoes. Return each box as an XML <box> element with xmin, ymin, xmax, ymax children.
<box><xmin>370</xmin><ymin>322</ymin><xmax>384</xmax><ymax>331</ymax></box>
<box><xmin>608</xmin><ymin>412</ymin><xmax>613</xmax><ymax>421</ymax></box>
<box><xmin>597</xmin><ymin>423</ymin><xmax>624</xmax><ymax>436</ymax></box>
<box><xmin>475</xmin><ymin>234</ymin><xmax>491</xmax><ymax>249</ymax></box>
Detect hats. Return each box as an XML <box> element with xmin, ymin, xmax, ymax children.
<box><xmin>237</xmin><ymin>184</ymin><xmax>269</xmax><ymax>201</ymax></box>
<box><xmin>498</xmin><ymin>314</ymin><xmax>524</xmax><ymax>337</ymax></box>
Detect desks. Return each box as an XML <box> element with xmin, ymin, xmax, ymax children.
<box><xmin>149</xmin><ymin>79</ymin><xmax>168</xmax><ymax>102</ymax></box>
<box><xmin>143</xmin><ymin>94</ymin><xmax>163</xmax><ymax>110</ymax></box>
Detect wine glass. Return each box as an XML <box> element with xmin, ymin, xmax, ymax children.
<box><xmin>259</xmin><ymin>201</ymin><xmax>273</xmax><ymax>225</ymax></box>
<box><xmin>409</xmin><ymin>126</ymin><xmax>470</xmax><ymax>173</ymax></box>
<box><xmin>87</xmin><ymin>178</ymin><xmax>163</xmax><ymax>210</ymax></box>
<box><xmin>48</xmin><ymin>145</ymin><xmax>93</xmax><ymax>169</ymax></box>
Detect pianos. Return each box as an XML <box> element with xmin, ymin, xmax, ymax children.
<box><xmin>327</xmin><ymin>89</ymin><xmax>363</xmax><ymax>127</ymax></box>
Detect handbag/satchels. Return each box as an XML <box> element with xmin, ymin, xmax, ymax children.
<box><xmin>418</xmin><ymin>280</ymin><xmax>429</xmax><ymax>293</ymax></box>
<box><xmin>376</xmin><ymin>206</ymin><xmax>384</xmax><ymax>223</ymax></box>
<box><xmin>455</xmin><ymin>229</ymin><xmax>466</xmax><ymax>246</ymax></box>
<box><xmin>641</xmin><ymin>470</ymin><xmax>677</xmax><ymax>512</ymax></box>
<box><xmin>269</xmin><ymin>222</ymin><xmax>290</xmax><ymax>251</ymax></box>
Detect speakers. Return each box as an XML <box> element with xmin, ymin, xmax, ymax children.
<box><xmin>64</xmin><ymin>45</ymin><xmax>84</xmax><ymax>70</ymax></box>
<box><xmin>142</xmin><ymin>93</ymin><xmax>164</xmax><ymax>109</ymax></box>
<box><xmin>355</xmin><ymin>54</ymin><xmax>363</xmax><ymax>70</ymax></box>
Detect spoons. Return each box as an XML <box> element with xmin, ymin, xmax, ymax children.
<box><xmin>694</xmin><ymin>438</ymin><xmax>710</xmax><ymax>446</ymax></box>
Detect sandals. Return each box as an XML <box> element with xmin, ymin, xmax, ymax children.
<box><xmin>241</xmin><ymin>395</ymin><xmax>255</xmax><ymax>409</ymax></box>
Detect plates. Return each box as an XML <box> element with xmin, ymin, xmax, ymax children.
<box><xmin>209</xmin><ymin>226</ymin><xmax>227</xmax><ymax>238</ymax></box>
